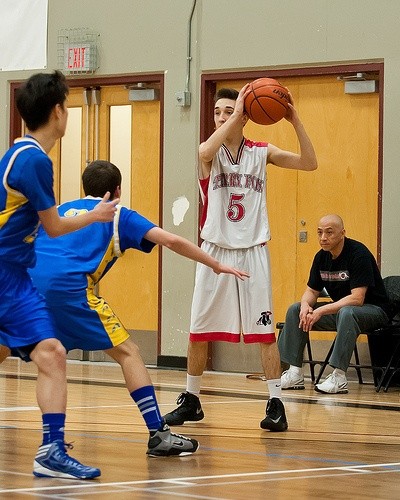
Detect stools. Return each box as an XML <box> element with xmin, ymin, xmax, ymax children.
<box><xmin>276</xmin><ymin>322</ymin><xmax>400</xmax><ymax>394</ymax></box>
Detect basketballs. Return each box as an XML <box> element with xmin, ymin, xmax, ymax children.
<box><xmin>243</xmin><ymin>77</ymin><xmax>291</xmax><ymax>126</ymax></box>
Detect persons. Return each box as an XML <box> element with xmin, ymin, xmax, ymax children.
<box><xmin>0</xmin><ymin>69</ymin><xmax>120</xmax><ymax>480</ymax></box>
<box><xmin>27</xmin><ymin>160</ymin><xmax>250</xmax><ymax>458</ymax></box>
<box><xmin>161</xmin><ymin>84</ymin><xmax>317</xmax><ymax>431</ymax></box>
<box><xmin>277</xmin><ymin>215</ymin><xmax>393</xmax><ymax>394</ymax></box>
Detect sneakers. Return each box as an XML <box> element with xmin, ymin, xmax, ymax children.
<box><xmin>146</xmin><ymin>425</ymin><xmax>198</xmax><ymax>457</ymax></box>
<box><xmin>260</xmin><ymin>397</ymin><xmax>289</xmax><ymax>432</ymax></box>
<box><xmin>32</xmin><ymin>440</ymin><xmax>101</xmax><ymax>480</ymax></box>
<box><xmin>280</xmin><ymin>370</ymin><xmax>305</xmax><ymax>389</ymax></box>
<box><xmin>315</xmin><ymin>373</ymin><xmax>349</xmax><ymax>393</ymax></box>
<box><xmin>162</xmin><ymin>392</ymin><xmax>205</xmax><ymax>425</ymax></box>
